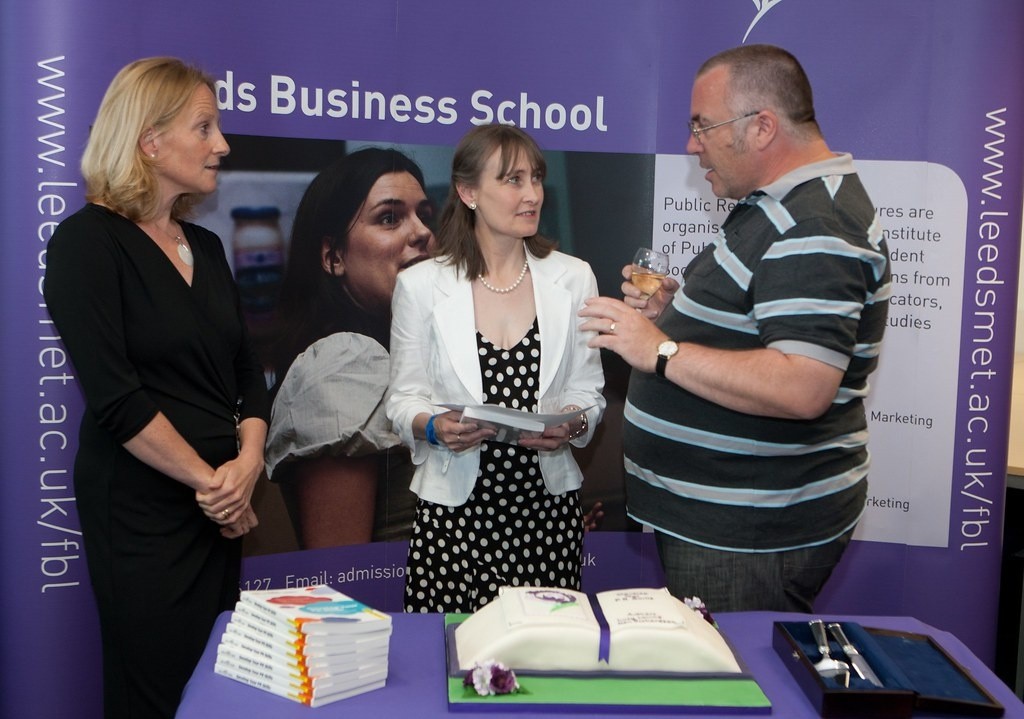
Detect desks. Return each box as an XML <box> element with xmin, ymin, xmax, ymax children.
<box><xmin>176</xmin><ymin>612</ymin><xmax>1024</xmax><ymax>719</ymax></box>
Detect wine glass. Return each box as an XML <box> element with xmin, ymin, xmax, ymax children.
<box><xmin>630</xmin><ymin>248</ymin><xmax>670</xmax><ymax>301</ymax></box>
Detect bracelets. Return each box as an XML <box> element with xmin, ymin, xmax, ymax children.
<box><xmin>424</xmin><ymin>414</ymin><xmax>439</xmax><ymax>446</ymax></box>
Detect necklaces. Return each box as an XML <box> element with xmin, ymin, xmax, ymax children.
<box><xmin>477</xmin><ymin>259</ymin><xmax>529</xmax><ymax>293</ymax></box>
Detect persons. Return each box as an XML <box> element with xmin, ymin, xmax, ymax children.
<box><xmin>257</xmin><ymin>146</ymin><xmax>607</xmax><ymax>551</ymax></box>
<box><xmin>578</xmin><ymin>45</ymin><xmax>894</xmax><ymax>612</ymax></box>
<box><xmin>41</xmin><ymin>57</ymin><xmax>272</xmax><ymax>719</ymax></box>
<box><xmin>383</xmin><ymin>123</ymin><xmax>609</xmax><ymax>616</ymax></box>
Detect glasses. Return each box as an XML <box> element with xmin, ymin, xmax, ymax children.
<box><xmin>687</xmin><ymin>111</ymin><xmax>760</xmax><ymax>145</ymax></box>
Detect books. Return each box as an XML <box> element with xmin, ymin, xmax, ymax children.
<box><xmin>213</xmin><ymin>583</ymin><xmax>393</xmax><ymax>709</ymax></box>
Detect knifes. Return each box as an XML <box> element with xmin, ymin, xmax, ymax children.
<box><xmin>829</xmin><ymin>621</ymin><xmax>881</xmax><ymax>688</ymax></box>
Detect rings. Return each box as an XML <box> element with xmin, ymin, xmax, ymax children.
<box><xmin>456</xmin><ymin>433</ymin><xmax>461</xmax><ymax>443</ymax></box>
<box><xmin>222</xmin><ymin>509</ymin><xmax>230</xmax><ymax>519</ymax></box>
<box><xmin>609</xmin><ymin>321</ymin><xmax>618</xmax><ymax>334</ymax></box>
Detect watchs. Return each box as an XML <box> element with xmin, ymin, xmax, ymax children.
<box><xmin>563</xmin><ymin>406</ymin><xmax>588</xmax><ymax>439</ymax></box>
<box><xmin>655</xmin><ymin>339</ymin><xmax>680</xmax><ymax>378</ymax></box>
